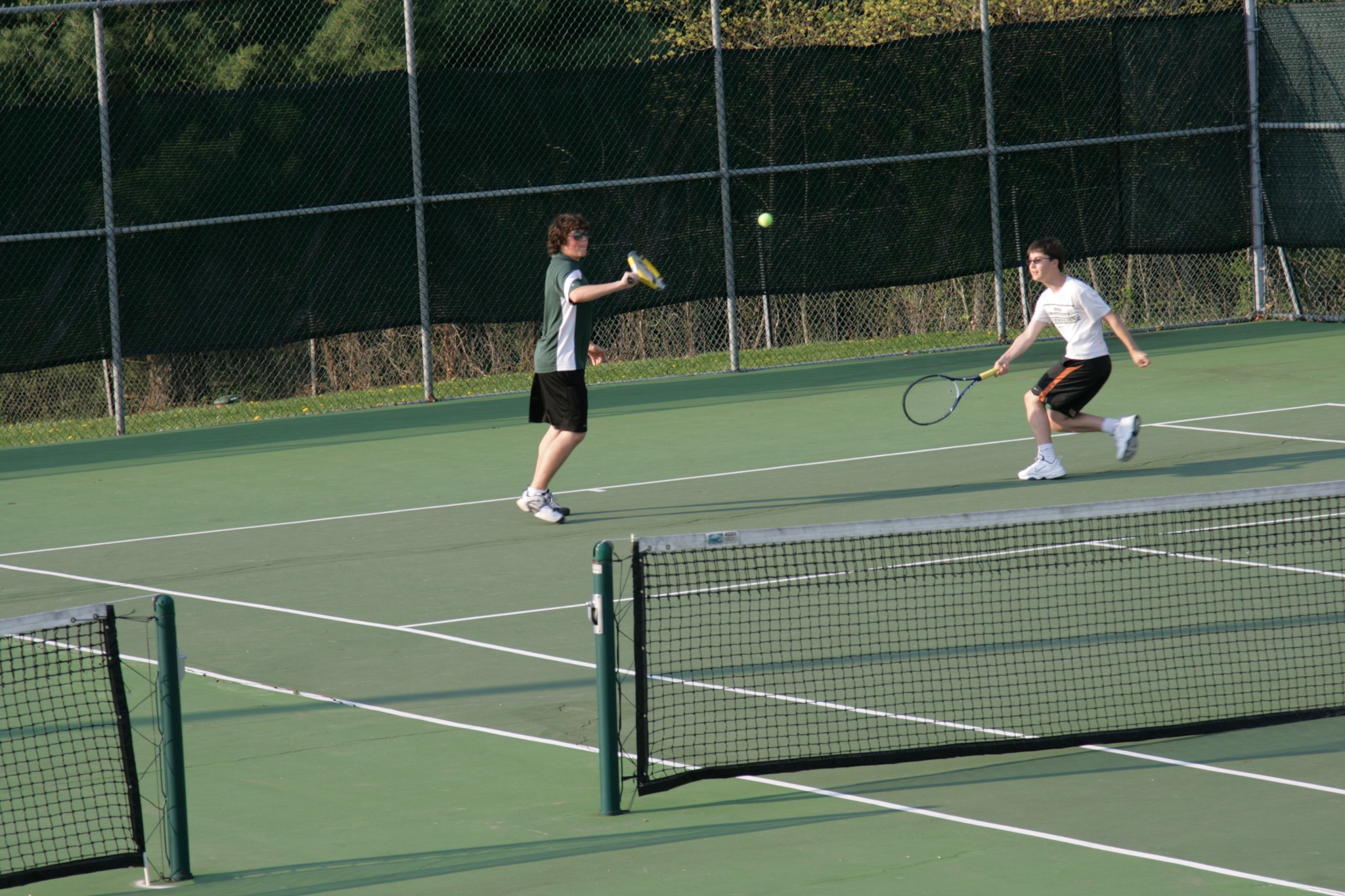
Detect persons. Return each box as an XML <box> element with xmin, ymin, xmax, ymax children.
<box><xmin>995</xmin><ymin>237</ymin><xmax>1151</xmax><ymax>480</ymax></box>
<box><xmin>514</xmin><ymin>214</ymin><xmax>642</xmax><ymax>523</ymax></box>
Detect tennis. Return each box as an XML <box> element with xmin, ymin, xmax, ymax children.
<box><xmin>757</xmin><ymin>212</ymin><xmax>773</xmax><ymax>228</ymax></box>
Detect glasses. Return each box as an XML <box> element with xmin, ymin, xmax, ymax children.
<box><xmin>562</xmin><ymin>231</ymin><xmax>590</xmax><ymax>241</ymax></box>
<box><xmin>1026</xmin><ymin>257</ymin><xmax>1055</xmax><ymax>265</ymax></box>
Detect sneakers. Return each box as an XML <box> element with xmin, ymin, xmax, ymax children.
<box><xmin>1112</xmin><ymin>413</ymin><xmax>1141</xmax><ymax>461</ymax></box>
<box><xmin>543</xmin><ymin>489</ymin><xmax>570</xmax><ymax>516</ymax></box>
<box><xmin>1017</xmin><ymin>455</ymin><xmax>1067</xmax><ymax>480</ymax></box>
<box><xmin>516</xmin><ymin>489</ymin><xmax>564</xmax><ymax>523</ymax></box>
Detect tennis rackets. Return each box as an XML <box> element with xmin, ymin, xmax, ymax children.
<box><xmin>627</xmin><ymin>250</ymin><xmax>666</xmax><ymax>292</ymax></box>
<box><xmin>902</xmin><ymin>363</ymin><xmax>1006</xmax><ymax>426</ymax></box>
